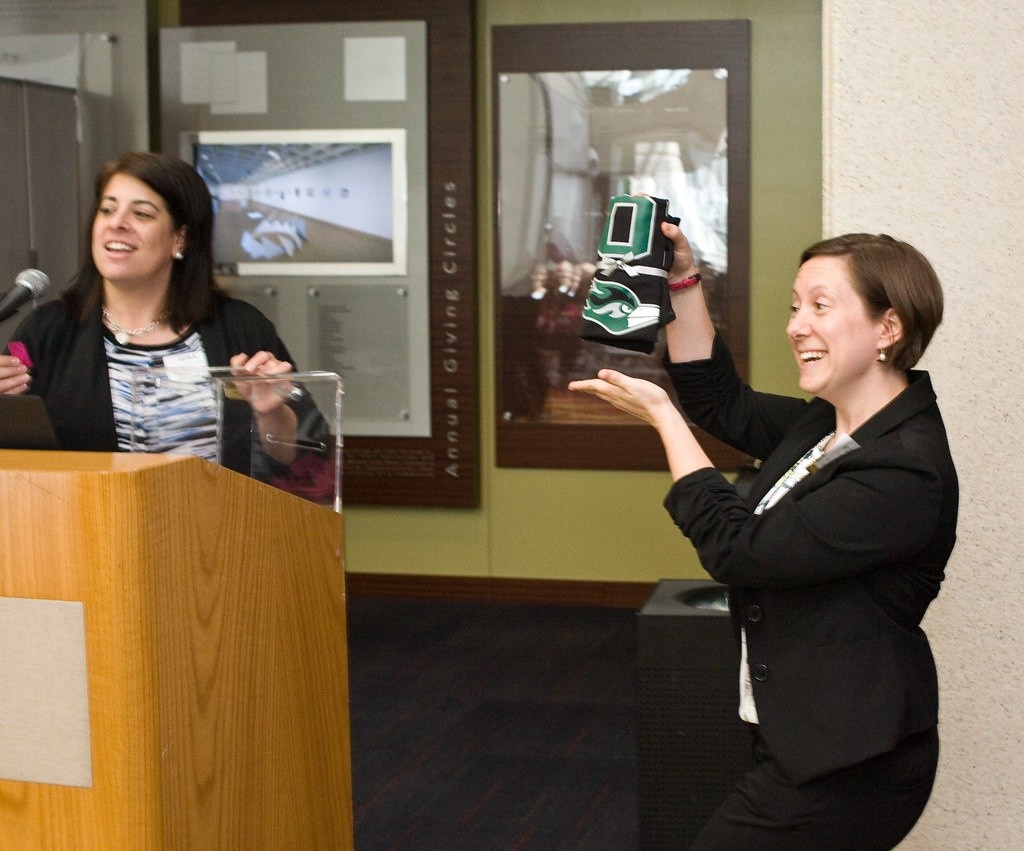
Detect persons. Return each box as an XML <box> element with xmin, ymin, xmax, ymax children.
<box><xmin>0</xmin><ymin>152</ymin><xmax>336</xmax><ymax>481</ymax></box>
<box><xmin>569</xmin><ymin>222</ymin><xmax>960</xmax><ymax>851</ymax></box>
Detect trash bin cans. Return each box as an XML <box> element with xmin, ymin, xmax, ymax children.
<box><xmin>633</xmin><ymin>577</ymin><xmax>753</xmax><ymax>851</ymax></box>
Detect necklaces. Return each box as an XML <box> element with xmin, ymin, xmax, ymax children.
<box><xmin>102</xmin><ymin>307</ymin><xmax>159</xmax><ymax>337</ymax></box>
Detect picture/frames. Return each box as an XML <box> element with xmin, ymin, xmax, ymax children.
<box><xmin>492</xmin><ymin>19</ymin><xmax>750</xmax><ymax>471</ymax></box>
<box><xmin>144</xmin><ymin>0</ymin><xmax>481</xmax><ymax>512</ymax></box>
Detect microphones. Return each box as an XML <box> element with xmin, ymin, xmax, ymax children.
<box><xmin>0</xmin><ymin>269</ymin><xmax>50</xmax><ymax>324</ymax></box>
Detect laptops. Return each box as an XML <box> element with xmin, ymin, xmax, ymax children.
<box><xmin>0</xmin><ymin>394</ymin><xmax>60</xmax><ymax>452</ymax></box>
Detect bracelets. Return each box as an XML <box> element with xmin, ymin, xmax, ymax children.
<box><xmin>669</xmin><ymin>272</ymin><xmax>702</xmax><ymax>290</ymax></box>
